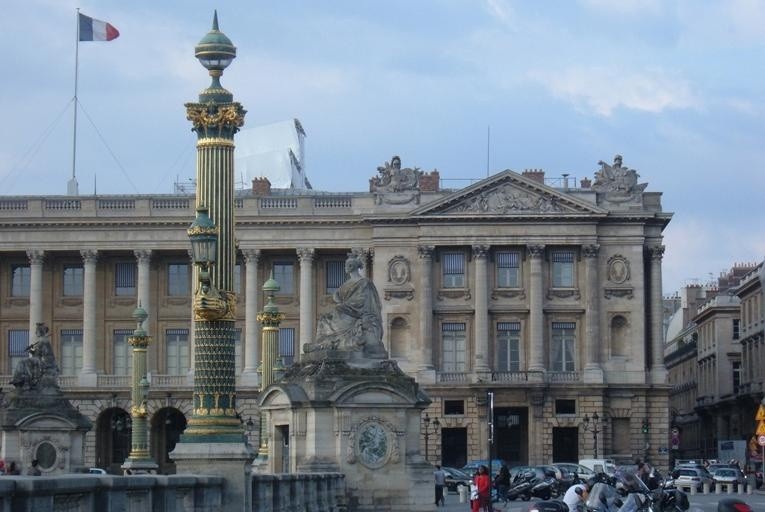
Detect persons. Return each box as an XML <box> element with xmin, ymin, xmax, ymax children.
<box><xmin>597</xmin><ymin>155</ymin><xmax>640</xmax><ymax>191</ymax></box>
<box><xmin>563</xmin><ymin>458</ymin><xmax>664</xmax><ymax>512</ymax></box>
<box><xmin>316</xmin><ymin>256</ymin><xmax>383</xmax><ymax>355</ymax></box>
<box><xmin>470</xmin><ymin>464</ymin><xmax>511</xmax><ymax>512</ymax></box>
<box><xmin>457</xmin><ymin>186</ymin><xmax>560</xmax><ymax>212</ymax></box>
<box><xmin>381</xmin><ymin>155</ymin><xmax>402</xmax><ymax>190</ymax></box>
<box><xmin>434</xmin><ymin>464</ymin><xmax>446</xmax><ymax>507</ymax></box>
<box><xmin>5</xmin><ymin>462</ymin><xmax>21</xmax><ymax>475</ymax></box>
<box><xmin>9</xmin><ymin>322</ymin><xmax>59</xmax><ymax>389</ymax></box>
<box><xmin>362</xmin><ymin>427</ymin><xmax>386</xmax><ymax>458</ymax></box>
<box><xmin>27</xmin><ymin>461</ymin><xmax>42</xmax><ymax>476</ymax></box>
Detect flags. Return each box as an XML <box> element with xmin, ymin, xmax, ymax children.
<box><xmin>79</xmin><ymin>12</ymin><xmax>120</xmax><ymax>41</ymax></box>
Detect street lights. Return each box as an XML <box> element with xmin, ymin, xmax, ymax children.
<box><xmin>423</xmin><ymin>411</ymin><xmax>430</xmax><ymax>461</ymax></box>
<box><xmin>590</xmin><ymin>410</ymin><xmax>600</xmax><ymax>459</ymax></box>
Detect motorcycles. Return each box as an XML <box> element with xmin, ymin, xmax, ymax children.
<box><xmin>528</xmin><ymin>476</ymin><xmax>561</xmax><ymax>500</ymax></box>
<box><xmin>528</xmin><ymin>464</ymin><xmax>691</xmax><ymax>511</ymax></box>
<box><xmin>491</xmin><ymin>469</ymin><xmax>535</xmax><ymax>501</ymax></box>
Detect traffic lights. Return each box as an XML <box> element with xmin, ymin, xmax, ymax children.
<box><xmin>641</xmin><ymin>416</ymin><xmax>649</xmax><ymax>433</ymax></box>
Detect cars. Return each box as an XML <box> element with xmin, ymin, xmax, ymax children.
<box><xmin>670</xmin><ymin>463</ymin><xmax>715</xmax><ymax>493</ymax></box>
<box><xmin>441</xmin><ymin>457</ymin><xmax>623</xmax><ymax>489</ymax></box>
<box><xmin>707</xmin><ymin>463</ymin><xmax>739</xmax><ymax>489</ymax></box>
<box><xmin>712</xmin><ymin>468</ymin><xmax>746</xmax><ymax>493</ymax></box>
